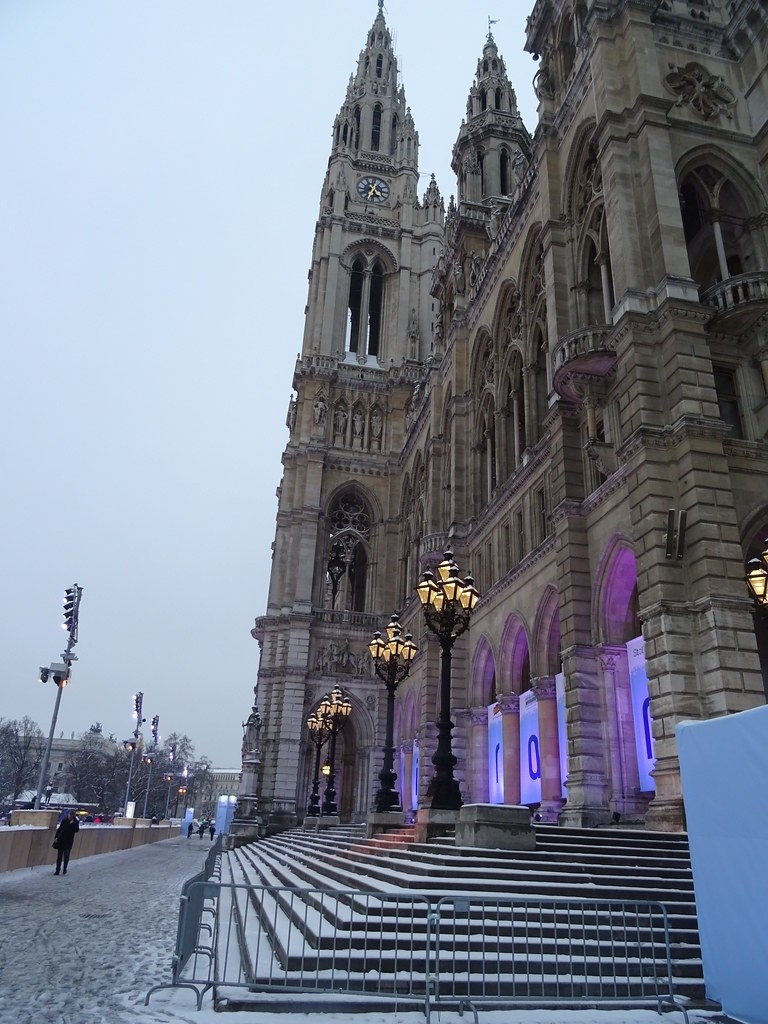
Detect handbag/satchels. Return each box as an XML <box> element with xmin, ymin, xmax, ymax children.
<box><xmin>52</xmin><ymin>839</ymin><xmax>58</xmax><ymax>850</ymax></box>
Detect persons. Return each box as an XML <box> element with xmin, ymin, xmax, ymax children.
<box><xmin>54</xmin><ymin>809</ymin><xmax>80</xmax><ymax>875</ymax></box>
<box><xmin>76</xmin><ymin>812</ymin><xmax>114</xmax><ymax>823</ymax></box>
<box><xmin>152</xmin><ymin>813</ymin><xmax>160</xmax><ymax>825</ymax></box>
<box><xmin>187</xmin><ymin>822</ymin><xmax>193</xmax><ymax>838</ymax></box>
<box><xmin>199</xmin><ymin>824</ymin><xmax>205</xmax><ymax>839</ymax></box>
<box><xmin>210</xmin><ymin>825</ymin><xmax>216</xmax><ymax>841</ymax></box>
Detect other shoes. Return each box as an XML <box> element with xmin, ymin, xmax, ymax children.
<box><xmin>53</xmin><ymin>870</ymin><xmax>59</xmax><ymax>875</ymax></box>
<box><xmin>63</xmin><ymin>870</ymin><xmax>67</xmax><ymax>874</ymax></box>
